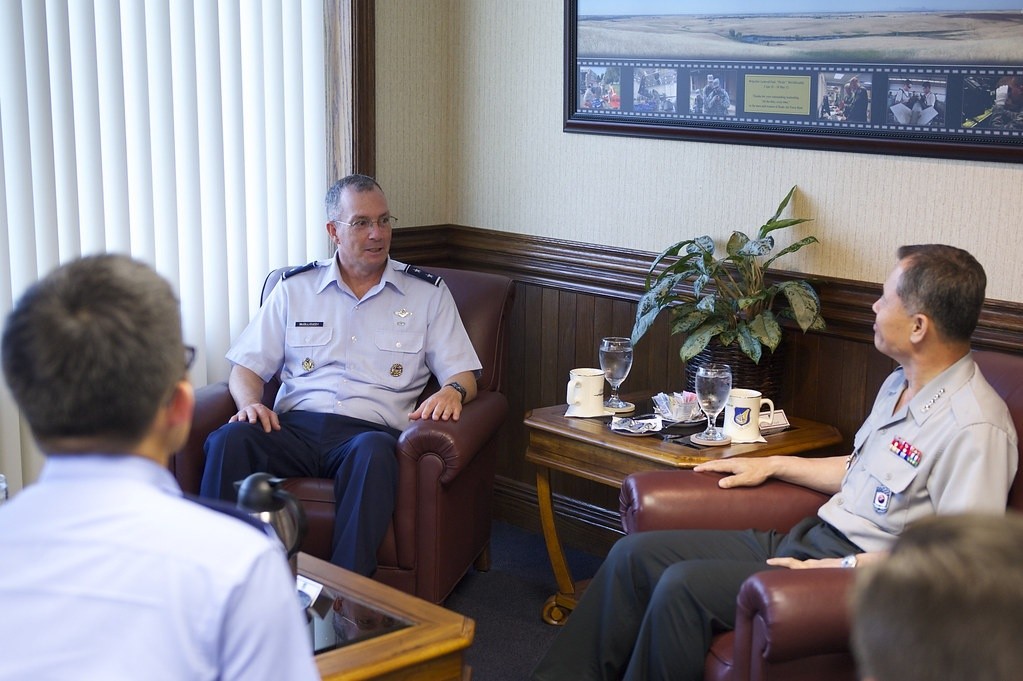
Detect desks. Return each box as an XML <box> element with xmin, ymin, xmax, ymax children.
<box><xmin>288</xmin><ymin>526</ymin><xmax>475</xmax><ymax>681</ymax></box>
<box><xmin>520</xmin><ymin>407</ymin><xmax>841</xmax><ymax>629</ymax></box>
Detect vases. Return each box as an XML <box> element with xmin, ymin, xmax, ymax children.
<box><xmin>683</xmin><ymin>335</ymin><xmax>772</xmax><ymax>403</ymax></box>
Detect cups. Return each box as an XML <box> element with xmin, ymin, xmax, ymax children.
<box><xmin>722</xmin><ymin>389</ymin><xmax>774</xmax><ymax>441</ymax></box>
<box><xmin>0</xmin><ymin>473</ymin><xmax>7</xmax><ymax>504</ymax></box>
<box><xmin>567</xmin><ymin>368</ymin><xmax>605</xmax><ymax>415</ymax></box>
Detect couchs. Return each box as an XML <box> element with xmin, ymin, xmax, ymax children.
<box><xmin>169</xmin><ymin>270</ymin><xmax>512</xmax><ymax>605</ymax></box>
<box><xmin>619</xmin><ymin>351</ymin><xmax>1023</xmax><ymax>681</ymax></box>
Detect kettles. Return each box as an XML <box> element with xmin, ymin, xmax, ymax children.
<box><xmin>233</xmin><ymin>471</ymin><xmax>303</xmax><ymax>587</ymax></box>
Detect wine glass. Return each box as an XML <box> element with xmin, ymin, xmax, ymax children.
<box><xmin>695</xmin><ymin>363</ymin><xmax>731</xmax><ymax>442</ymax></box>
<box><xmin>599</xmin><ymin>337</ymin><xmax>633</xmax><ymax>408</ymax></box>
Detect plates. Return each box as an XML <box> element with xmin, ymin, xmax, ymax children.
<box><xmin>608</xmin><ymin>413</ymin><xmax>662</xmax><ymax>436</ymax></box>
<box><xmin>658</xmin><ymin>417</ymin><xmax>707</xmax><ymax>426</ymax></box>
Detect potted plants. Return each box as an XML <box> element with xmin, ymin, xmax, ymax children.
<box><xmin>628</xmin><ymin>184</ymin><xmax>831</xmax><ymax>411</ymax></box>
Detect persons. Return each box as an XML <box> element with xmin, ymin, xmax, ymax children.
<box><xmin>888</xmin><ymin>79</ymin><xmax>935</xmax><ymax>126</ymax></box>
<box><xmin>581</xmin><ymin>69</ymin><xmax>620</xmax><ymax>111</ymax></box>
<box><xmin>331</xmin><ymin>596</ymin><xmax>409</xmax><ymax>644</ymax></box>
<box><xmin>0</xmin><ymin>251</ymin><xmax>323</xmax><ymax>681</ymax></box>
<box><xmin>653</xmin><ymin>90</ymin><xmax>676</xmax><ymax>111</ymax></box>
<box><xmin>834</xmin><ymin>76</ymin><xmax>868</xmax><ymax>123</ymax></box>
<box><xmin>198</xmin><ymin>171</ymin><xmax>483</xmax><ymax>577</ymax></box>
<box><xmin>531</xmin><ymin>243</ymin><xmax>1023</xmax><ymax>681</ymax></box>
<box><xmin>694</xmin><ymin>74</ymin><xmax>731</xmax><ymax>115</ymax></box>
<box><xmin>990</xmin><ymin>75</ymin><xmax>1023</xmax><ymax>129</ymax></box>
<box><xmin>847</xmin><ymin>500</ymin><xmax>1022</xmax><ymax>680</ymax></box>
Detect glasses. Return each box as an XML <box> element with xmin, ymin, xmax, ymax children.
<box><xmin>184</xmin><ymin>344</ymin><xmax>195</xmax><ymax>372</ymax></box>
<box><xmin>337</xmin><ymin>216</ymin><xmax>398</xmax><ymax>231</ymax></box>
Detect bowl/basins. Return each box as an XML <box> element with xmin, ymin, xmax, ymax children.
<box><xmin>757</xmin><ymin>409</ymin><xmax>790</xmax><ymax>435</ymax></box>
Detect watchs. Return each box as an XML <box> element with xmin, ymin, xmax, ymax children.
<box><xmin>444</xmin><ymin>381</ymin><xmax>467</xmax><ymax>402</ymax></box>
<box><xmin>841</xmin><ymin>552</ymin><xmax>857</xmax><ymax>568</ymax></box>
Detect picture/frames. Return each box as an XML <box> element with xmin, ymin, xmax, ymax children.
<box><xmin>562</xmin><ymin>0</ymin><xmax>1023</xmax><ymax>166</ymax></box>
